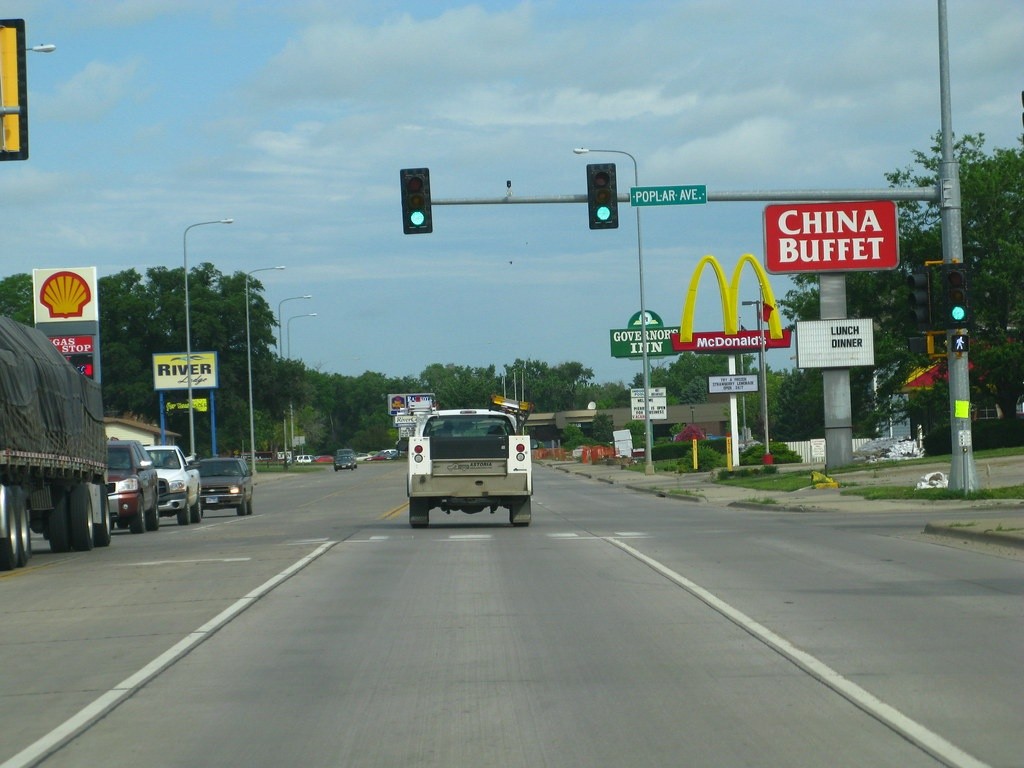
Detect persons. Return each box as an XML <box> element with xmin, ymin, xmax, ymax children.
<box><xmin>167</xmin><ymin>456</ymin><xmax>175</xmax><ymax>467</ymax></box>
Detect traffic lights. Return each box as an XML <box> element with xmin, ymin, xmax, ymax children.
<box><xmin>951</xmin><ymin>335</ymin><xmax>969</xmax><ymax>353</ymax></box>
<box><xmin>907</xmin><ymin>269</ymin><xmax>934</xmax><ymax>328</ymax></box>
<box><xmin>929</xmin><ymin>330</ymin><xmax>950</xmax><ymax>356</ymax></box>
<box><xmin>586</xmin><ymin>162</ymin><xmax>619</xmax><ymax>230</ymax></box>
<box><xmin>907</xmin><ymin>336</ymin><xmax>927</xmax><ymax>353</ymax></box>
<box><xmin>399</xmin><ymin>167</ymin><xmax>433</xmax><ymax>235</ymax></box>
<box><xmin>941</xmin><ymin>268</ymin><xmax>970</xmax><ymax>323</ymax></box>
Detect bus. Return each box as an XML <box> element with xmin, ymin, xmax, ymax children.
<box><xmin>239</xmin><ymin>451</ymin><xmax>273</xmax><ymax>464</ymax></box>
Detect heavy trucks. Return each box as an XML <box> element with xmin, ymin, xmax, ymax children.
<box><xmin>0</xmin><ymin>350</ymin><xmax>113</xmax><ymax>572</ymax></box>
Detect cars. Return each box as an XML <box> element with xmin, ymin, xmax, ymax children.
<box><xmin>312</xmin><ymin>455</ymin><xmax>334</xmax><ymax>463</ymax></box>
<box><xmin>334</xmin><ymin>449</ymin><xmax>358</xmax><ymax>471</ymax></box>
<box><xmin>198</xmin><ymin>457</ymin><xmax>257</xmax><ymax>514</ymax></box>
<box><xmin>355</xmin><ymin>449</ymin><xmax>399</xmax><ymax>461</ymax></box>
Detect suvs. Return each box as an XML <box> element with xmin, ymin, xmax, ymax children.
<box><xmin>104</xmin><ymin>439</ymin><xmax>162</xmax><ymax>533</ymax></box>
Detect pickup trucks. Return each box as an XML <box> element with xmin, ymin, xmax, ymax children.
<box><xmin>406</xmin><ymin>408</ymin><xmax>537</xmax><ymax>527</ymax></box>
<box><xmin>143</xmin><ymin>445</ymin><xmax>207</xmax><ymax>524</ymax></box>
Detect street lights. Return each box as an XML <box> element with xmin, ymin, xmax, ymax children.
<box><xmin>183</xmin><ymin>218</ymin><xmax>237</xmax><ymax>462</ymax></box>
<box><xmin>285</xmin><ymin>313</ymin><xmax>316</xmax><ymax>456</ymax></box>
<box><xmin>276</xmin><ymin>294</ymin><xmax>316</xmax><ymax>470</ymax></box>
<box><xmin>246</xmin><ymin>266</ymin><xmax>287</xmax><ymax>484</ymax></box>
<box><xmin>572</xmin><ymin>148</ymin><xmax>657</xmax><ymax>476</ymax></box>
<box><xmin>741</xmin><ymin>300</ymin><xmax>769</xmax><ymax>442</ymax></box>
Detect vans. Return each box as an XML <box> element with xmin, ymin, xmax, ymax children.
<box><xmin>293</xmin><ymin>455</ymin><xmax>315</xmax><ymax>464</ymax></box>
